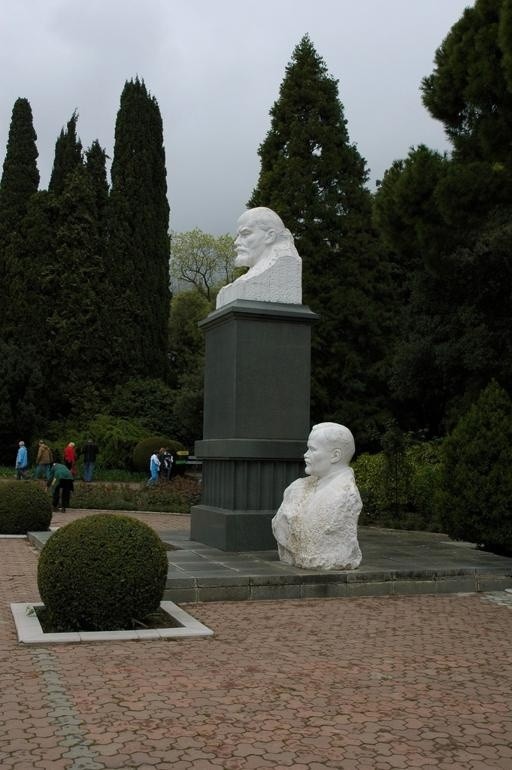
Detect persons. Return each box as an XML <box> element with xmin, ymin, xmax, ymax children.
<box><xmin>148</xmin><ymin>450</ymin><xmax>162</xmax><ymax>482</ymax></box>
<box><xmin>16</xmin><ymin>440</ymin><xmax>31</xmax><ymax>480</ymax></box>
<box><xmin>216</xmin><ymin>207</ymin><xmax>303</xmax><ymax>309</ymax></box>
<box><xmin>159</xmin><ymin>447</ymin><xmax>174</xmax><ymax>480</ymax></box>
<box><xmin>80</xmin><ymin>439</ymin><xmax>99</xmax><ymax>482</ymax></box>
<box><xmin>44</xmin><ymin>462</ymin><xmax>73</xmax><ymax>513</ymax></box>
<box><xmin>34</xmin><ymin>440</ymin><xmax>49</xmax><ymax>477</ymax></box>
<box><xmin>64</xmin><ymin>442</ymin><xmax>80</xmax><ymax>479</ymax></box>
<box><xmin>268</xmin><ymin>420</ymin><xmax>365</xmax><ymax>569</ymax></box>
